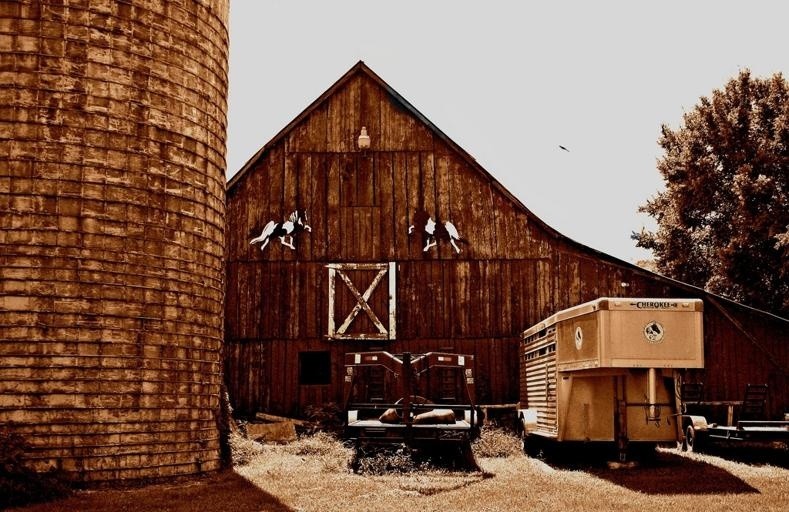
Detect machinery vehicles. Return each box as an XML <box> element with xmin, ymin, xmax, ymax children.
<box><xmin>343</xmin><ymin>352</ymin><xmax>485</xmax><ymax>457</ymax></box>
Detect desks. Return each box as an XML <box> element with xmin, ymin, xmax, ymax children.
<box><xmin>682</xmin><ymin>401</ymin><xmax>749</xmax><ymax>427</ymax></box>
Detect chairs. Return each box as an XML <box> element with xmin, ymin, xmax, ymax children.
<box><xmin>739</xmin><ymin>384</ymin><xmax>768</xmax><ymax>421</ymax></box>
<box><xmin>682</xmin><ymin>382</ymin><xmax>704</xmax><ymax>414</ymax></box>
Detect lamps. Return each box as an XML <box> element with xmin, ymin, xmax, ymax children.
<box><xmin>357</xmin><ymin>126</ymin><xmax>371</xmax><ymax>150</ymax></box>
